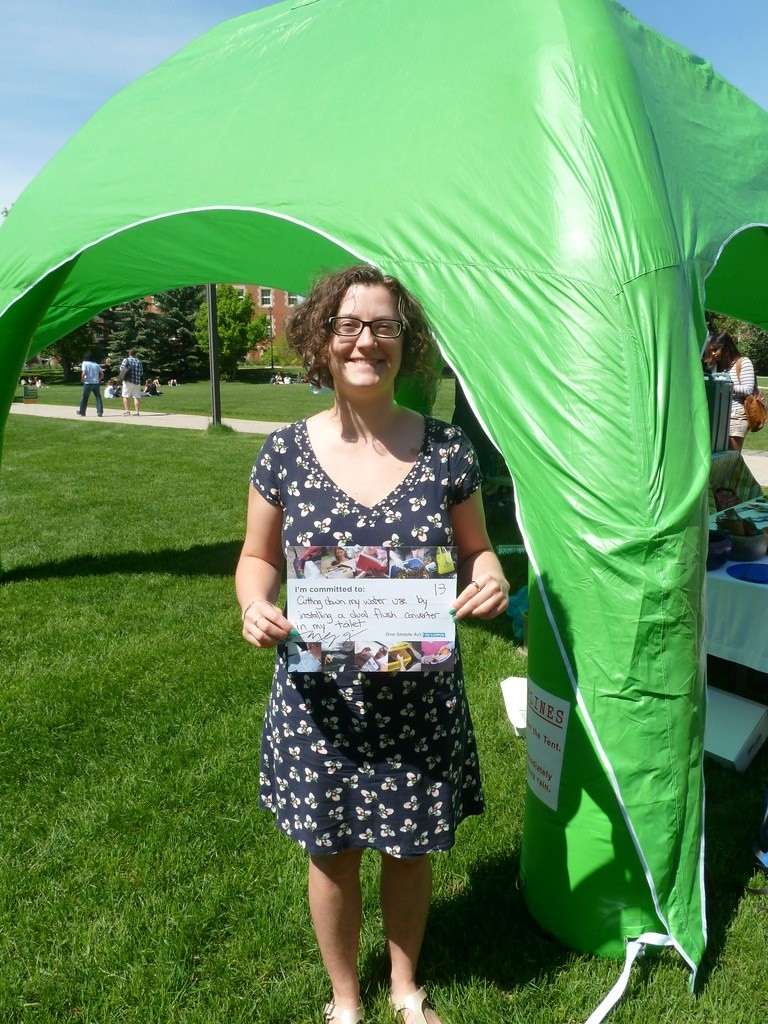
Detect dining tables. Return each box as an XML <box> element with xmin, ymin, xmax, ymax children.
<box><xmin>705</xmin><ymin>493</ymin><xmax>768</xmax><ymax>673</ymax></box>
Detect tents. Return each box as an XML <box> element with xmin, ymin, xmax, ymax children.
<box><xmin>0</xmin><ymin>0</ymin><xmax>766</xmax><ymax>997</ymax></box>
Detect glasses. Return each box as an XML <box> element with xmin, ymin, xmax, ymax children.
<box><xmin>329</xmin><ymin>317</ymin><xmax>406</xmax><ymax>339</ymax></box>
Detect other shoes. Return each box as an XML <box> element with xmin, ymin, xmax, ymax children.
<box><xmin>97</xmin><ymin>413</ymin><xmax>102</xmax><ymax>417</ymax></box>
<box><xmin>123</xmin><ymin>412</ymin><xmax>130</xmax><ymax>415</ymax></box>
<box><xmin>133</xmin><ymin>413</ymin><xmax>139</xmax><ymax>416</ymax></box>
<box><xmin>77</xmin><ymin>411</ymin><xmax>85</xmax><ymax>416</ymax></box>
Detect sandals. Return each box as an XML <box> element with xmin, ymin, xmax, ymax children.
<box><xmin>389</xmin><ymin>985</ymin><xmax>430</xmax><ymax>1023</ymax></box>
<box><xmin>323</xmin><ymin>994</ymin><xmax>365</xmax><ymax>1024</ymax></box>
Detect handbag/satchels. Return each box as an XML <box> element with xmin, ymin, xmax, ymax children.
<box><xmin>744</xmin><ymin>389</ymin><xmax>766</xmax><ymax>432</ymax></box>
<box><xmin>120</xmin><ymin>365</ymin><xmax>132</xmax><ymax>382</ymax></box>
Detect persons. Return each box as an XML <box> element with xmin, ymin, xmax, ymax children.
<box><xmin>235</xmin><ymin>262</ymin><xmax>511</xmax><ymax>1023</ymax></box>
<box><xmin>19</xmin><ymin>371</ymin><xmax>312</xmax><ymax>398</ymax></box>
<box><xmin>76</xmin><ymin>351</ymin><xmax>103</xmax><ymax>417</ymax></box>
<box><xmin>354</xmin><ymin>645</ymin><xmax>388</xmax><ymax>672</ymax></box>
<box><xmin>709</xmin><ymin>333</ymin><xmax>755</xmax><ymax>453</ymax></box>
<box><xmin>321</xmin><ymin>546</ymin><xmax>388</xmax><ymax>579</ymax></box>
<box><xmin>117</xmin><ymin>349</ymin><xmax>144</xmax><ymax>416</ymax></box>
